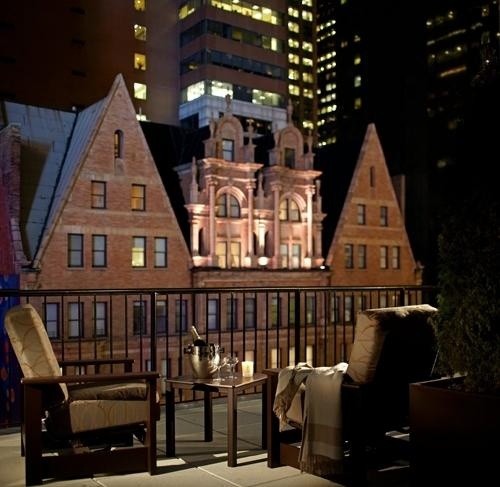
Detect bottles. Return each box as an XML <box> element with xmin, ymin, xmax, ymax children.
<box><xmin>189</xmin><ymin>325</ymin><xmax>207</xmax><ymax>346</ymax></box>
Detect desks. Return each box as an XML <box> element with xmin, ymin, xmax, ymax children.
<box><xmin>164</xmin><ymin>372</ymin><xmax>269</xmax><ymax>467</ymax></box>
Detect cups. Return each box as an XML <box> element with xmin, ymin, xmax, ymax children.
<box><xmin>242</xmin><ymin>361</ymin><xmax>254</xmax><ymax>377</ymax></box>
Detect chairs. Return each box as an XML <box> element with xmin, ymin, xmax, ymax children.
<box><xmin>4</xmin><ymin>303</ymin><xmax>160</xmax><ymax>486</ymax></box>
<box><xmin>262</xmin><ymin>303</ymin><xmax>439</xmax><ymax>487</ymax></box>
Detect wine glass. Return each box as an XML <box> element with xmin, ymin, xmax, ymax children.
<box><xmin>212</xmin><ymin>356</ymin><xmax>239</xmax><ymax>380</ymax></box>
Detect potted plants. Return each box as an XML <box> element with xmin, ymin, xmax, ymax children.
<box><xmin>408</xmin><ymin>232</ymin><xmax>500</xmax><ymax>487</ymax></box>
